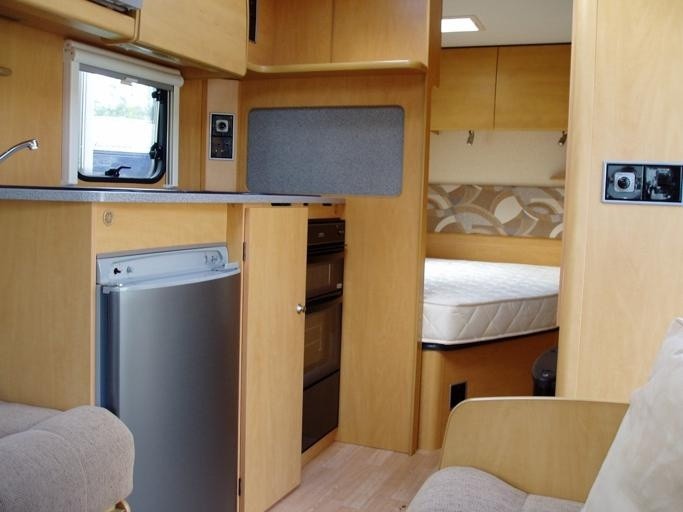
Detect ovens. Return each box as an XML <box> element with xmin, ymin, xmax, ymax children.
<box><xmin>303</xmin><ymin>218</ymin><xmax>345</xmax><ymax>453</ymax></box>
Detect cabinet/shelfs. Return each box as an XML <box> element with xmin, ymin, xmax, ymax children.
<box><xmin>491</xmin><ymin>42</ymin><xmax>571</xmax><ymax>134</ymax></box>
<box><xmin>428</xmin><ymin>45</ymin><xmax>498</xmax><ymax>132</ymax></box>
<box><xmin>224</xmin><ymin>202</ymin><xmax>311</xmax><ymax>511</ymax></box>
<box><xmin>98</xmin><ymin>1</ymin><xmax>249</xmax><ymax>81</ymax></box>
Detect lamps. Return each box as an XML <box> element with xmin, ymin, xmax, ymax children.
<box><xmin>465</xmin><ymin>131</ymin><xmax>476</xmax><ymax>146</ymax></box>
<box><xmin>557</xmin><ymin>131</ymin><xmax>567</xmax><ymax>146</ymax></box>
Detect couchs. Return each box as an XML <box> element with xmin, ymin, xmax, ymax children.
<box><xmin>405</xmin><ymin>317</ymin><xmax>682</xmax><ymax>509</ymax></box>
<box><xmin>0</xmin><ymin>404</ymin><xmax>135</xmax><ymax>510</ymax></box>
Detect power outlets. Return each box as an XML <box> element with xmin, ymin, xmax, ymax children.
<box><xmin>209</xmin><ymin>137</ymin><xmax>232</xmax><ymax>159</ymax></box>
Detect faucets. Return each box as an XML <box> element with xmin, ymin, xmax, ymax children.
<box><xmin>0</xmin><ymin>137</ymin><xmax>39</xmax><ymax>162</ymax></box>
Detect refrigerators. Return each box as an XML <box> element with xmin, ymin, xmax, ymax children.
<box><xmin>93</xmin><ymin>244</ymin><xmax>242</xmax><ymax>512</ymax></box>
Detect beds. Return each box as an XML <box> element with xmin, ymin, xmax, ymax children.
<box><xmin>419</xmin><ymin>256</ymin><xmax>561</xmax><ymax>452</ymax></box>
